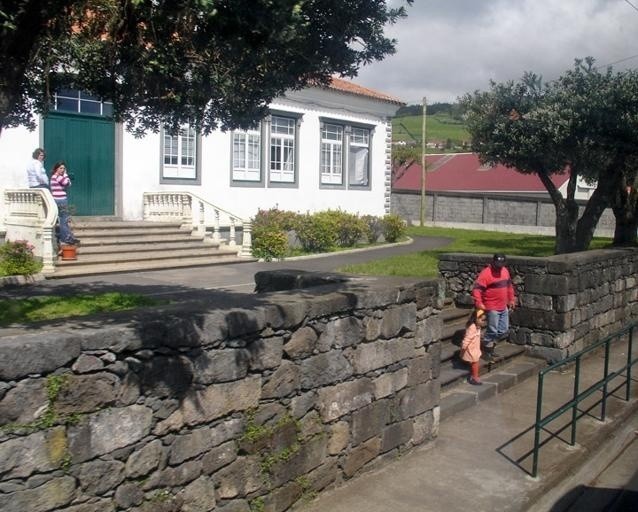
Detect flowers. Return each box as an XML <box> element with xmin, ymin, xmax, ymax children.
<box><xmin>60</xmin><ymin>204</ymin><xmax>80</xmax><ymax>245</ymax></box>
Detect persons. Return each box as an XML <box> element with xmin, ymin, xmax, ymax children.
<box><xmin>457</xmin><ymin>308</ymin><xmax>488</xmax><ymax>387</ymax></box>
<box><xmin>26</xmin><ymin>147</ymin><xmax>50</xmax><ymax>191</ymax></box>
<box><xmin>469</xmin><ymin>252</ymin><xmax>518</xmax><ymax>361</ymax></box>
<box><xmin>46</xmin><ymin>161</ymin><xmax>81</xmax><ymax>247</ymax></box>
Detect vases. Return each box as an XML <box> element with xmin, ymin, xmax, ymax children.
<box><xmin>61</xmin><ymin>244</ymin><xmax>77</xmax><ymax>260</ymax></box>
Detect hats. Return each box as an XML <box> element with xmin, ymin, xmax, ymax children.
<box><xmin>493</xmin><ymin>253</ymin><xmax>505</xmax><ymax>269</ymax></box>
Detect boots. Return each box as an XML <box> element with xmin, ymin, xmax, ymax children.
<box><xmin>482</xmin><ymin>345</ymin><xmax>499</xmax><ymax>363</ymax></box>
